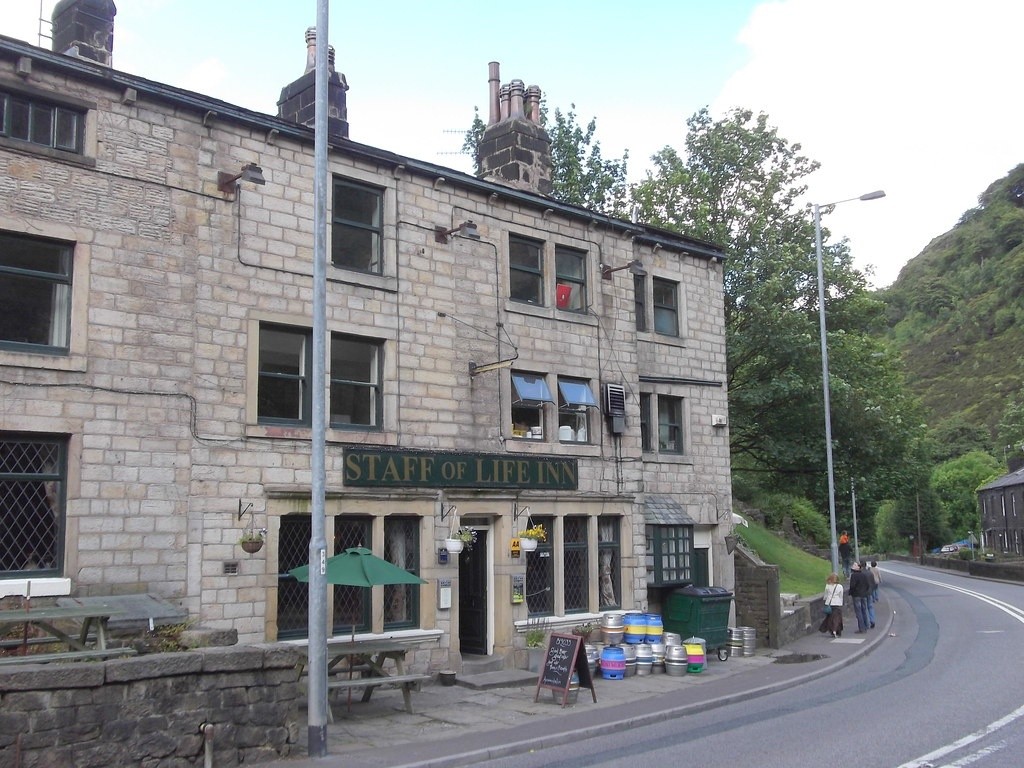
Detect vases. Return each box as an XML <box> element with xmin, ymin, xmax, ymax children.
<box><xmin>445</xmin><ymin>539</ymin><xmax>464</xmax><ymax>553</ymax></box>
<box><xmin>439</xmin><ymin>671</ymin><xmax>455</xmax><ymax>686</ymax></box>
<box><xmin>527</xmin><ymin>647</ymin><xmax>546</xmax><ymax>675</ymax></box>
<box><xmin>520</xmin><ymin>538</ymin><xmax>537</xmax><ymax>551</ymax></box>
<box><xmin>575</xmin><ymin>632</ymin><xmax>591</xmax><ymax>642</ymax></box>
<box><xmin>241</xmin><ymin>540</ymin><xmax>263</xmax><ymax>554</ymax></box>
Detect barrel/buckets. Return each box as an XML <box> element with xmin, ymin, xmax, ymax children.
<box><xmin>571</xmin><ymin>612</ymin><xmax>708</xmax><ymax>683</ymax></box>
<box><xmin>727</xmin><ymin>626</ymin><xmax>756</xmax><ymax>657</ymax></box>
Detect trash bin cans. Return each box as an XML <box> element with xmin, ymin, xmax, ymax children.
<box><xmin>661</xmin><ymin>584</ymin><xmax>734</xmax><ymax>661</ymax></box>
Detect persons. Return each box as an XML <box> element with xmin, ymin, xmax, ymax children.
<box><xmin>839</xmin><ymin>530</ymin><xmax>853</xmax><ymax>578</ymax></box>
<box><xmin>823</xmin><ymin>575</ymin><xmax>843</xmax><ymax>639</ymax></box>
<box><xmin>849</xmin><ymin>560</ymin><xmax>882</xmax><ymax>634</ymax></box>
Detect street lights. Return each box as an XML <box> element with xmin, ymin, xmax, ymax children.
<box><xmin>814</xmin><ymin>191</ymin><xmax>886</xmax><ymax>573</ymax></box>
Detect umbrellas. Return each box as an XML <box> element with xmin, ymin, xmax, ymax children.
<box><xmin>288</xmin><ymin>542</ymin><xmax>430</xmax><ymax>714</ymax></box>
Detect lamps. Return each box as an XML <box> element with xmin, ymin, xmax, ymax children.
<box><xmin>435</xmin><ymin>219</ymin><xmax>480</xmax><ymax>243</ymax></box>
<box><xmin>216</xmin><ymin>162</ymin><xmax>266</xmax><ymax>192</ymax></box>
<box><xmin>602</xmin><ymin>260</ymin><xmax>648</xmax><ymax>280</ymax></box>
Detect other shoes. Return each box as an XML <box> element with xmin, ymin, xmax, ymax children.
<box><xmin>832</xmin><ymin>631</ymin><xmax>836</xmax><ymax>639</ymax></box>
<box><xmin>854</xmin><ymin>628</ymin><xmax>867</xmax><ymax>634</ymax></box>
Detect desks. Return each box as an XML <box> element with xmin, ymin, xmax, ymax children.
<box><xmin>0</xmin><ymin>604</ymin><xmax>128</xmax><ymax>661</ymax></box>
<box><xmin>296</xmin><ymin>639</ymin><xmax>414</xmax><ymax>724</ymax></box>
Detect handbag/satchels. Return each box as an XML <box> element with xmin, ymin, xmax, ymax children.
<box><xmin>821</xmin><ymin>605</ymin><xmax>833</xmax><ymax>614</ymax></box>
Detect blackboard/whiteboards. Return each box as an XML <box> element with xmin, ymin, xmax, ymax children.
<box><xmin>539</xmin><ymin>635</ymin><xmax>590</xmax><ymax>688</ymax></box>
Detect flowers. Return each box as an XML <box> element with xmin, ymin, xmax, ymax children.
<box><xmin>525</xmin><ymin>615</ymin><xmax>553</xmax><ymax>648</ymax></box>
<box><xmin>236</xmin><ymin>526</ymin><xmax>268</xmax><ymax>544</ymax></box>
<box><xmin>518</xmin><ymin>524</ymin><xmax>548</xmax><ymax>542</ymax></box>
<box><xmin>572</xmin><ymin>622</ymin><xmax>594</xmax><ymax>634</ymax></box>
<box><xmin>447</xmin><ymin>526</ymin><xmax>477</xmax><ymax>551</ymax></box>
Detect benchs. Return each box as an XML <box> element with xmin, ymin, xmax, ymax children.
<box><xmin>0</xmin><ymin>647</ymin><xmax>139</xmax><ymax>666</ymax></box>
<box><xmin>301</xmin><ymin>664</ymin><xmax>371</xmax><ymax>676</ymax></box>
<box><xmin>0</xmin><ymin>633</ymin><xmax>97</xmax><ymax>648</ymax></box>
<box><xmin>328</xmin><ymin>673</ymin><xmax>432</xmax><ymax>689</ymax></box>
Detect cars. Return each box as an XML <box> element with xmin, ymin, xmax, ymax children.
<box><xmin>940</xmin><ymin>545</ymin><xmax>958</xmax><ymax>553</ymax></box>
